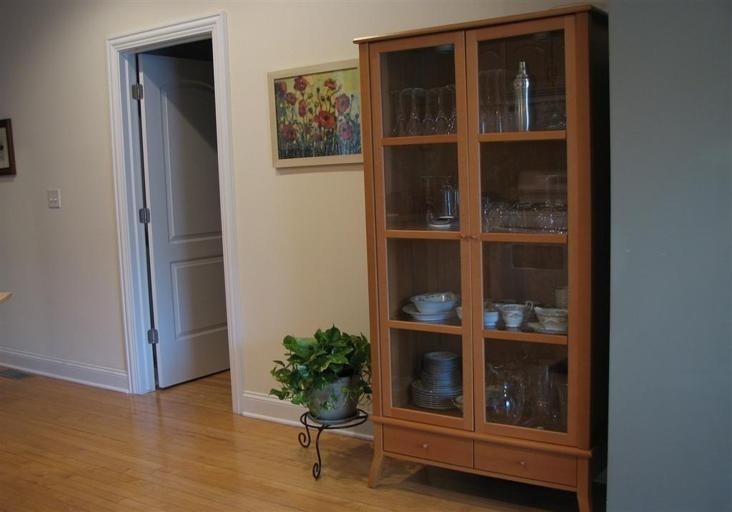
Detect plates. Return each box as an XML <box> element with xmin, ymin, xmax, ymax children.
<box><xmin>401</xmin><ymin>303</ymin><xmax>456</xmax><ymax>322</ymax></box>
<box><xmin>410</xmin><ymin>349</ymin><xmax>462</xmax><ymax>411</ymax></box>
<box><xmin>527</xmin><ymin>287</ymin><xmax>568</xmax><ymax>335</ymax></box>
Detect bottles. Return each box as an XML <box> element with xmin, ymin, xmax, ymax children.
<box><xmin>484</xmin><ymin>362</ymin><xmax>526</xmax><ymax>424</ymax></box>
<box><xmin>512</xmin><ymin>60</ymin><xmax>532</xmax><ymax>131</ymax></box>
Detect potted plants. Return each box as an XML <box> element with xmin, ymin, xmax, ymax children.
<box><xmin>267</xmin><ymin>323</ymin><xmax>371</xmax><ymax>425</ymax></box>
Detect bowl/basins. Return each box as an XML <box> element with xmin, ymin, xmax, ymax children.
<box><xmin>484</xmin><ymin>311</ymin><xmax>499</xmax><ymax>326</ymax></box>
<box><xmin>410</xmin><ymin>292</ymin><xmax>457</xmax><ymax>313</ymax></box>
<box><xmin>456</xmin><ymin>306</ymin><xmax>462</xmax><ymax>320</ymax></box>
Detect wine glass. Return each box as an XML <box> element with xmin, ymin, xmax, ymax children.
<box><xmin>419</xmin><ymin>175</ymin><xmax>459</xmax><ymax>227</ymax></box>
<box><xmin>390</xmin><ymin>67</ymin><xmax>516</xmax><ymax>138</ymax></box>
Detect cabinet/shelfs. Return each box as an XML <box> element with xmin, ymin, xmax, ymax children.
<box><xmin>352</xmin><ymin>4</ymin><xmax>609</xmax><ymax>512</ymax></box>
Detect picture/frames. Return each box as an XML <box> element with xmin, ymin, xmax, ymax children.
<box><xmin>266</xmin><ymin>57</ymin><xmax>364</xmax><ymax>169</ymax></box>
<box><xmin>0</xmin><ymin>118</ymin><xmax>17</xmax><ymax>176</ymax></box>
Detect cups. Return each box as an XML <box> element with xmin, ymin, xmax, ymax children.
<box><xmin>481</xmin><ymin>195</ymin><xmax>567</xmax><ymax>233</ymax></box>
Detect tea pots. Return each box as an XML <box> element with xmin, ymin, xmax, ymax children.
<box><xmin>494</xmin><ymin>300</ymin><xmax>534</xmax><ymax>328</ymax></box>
